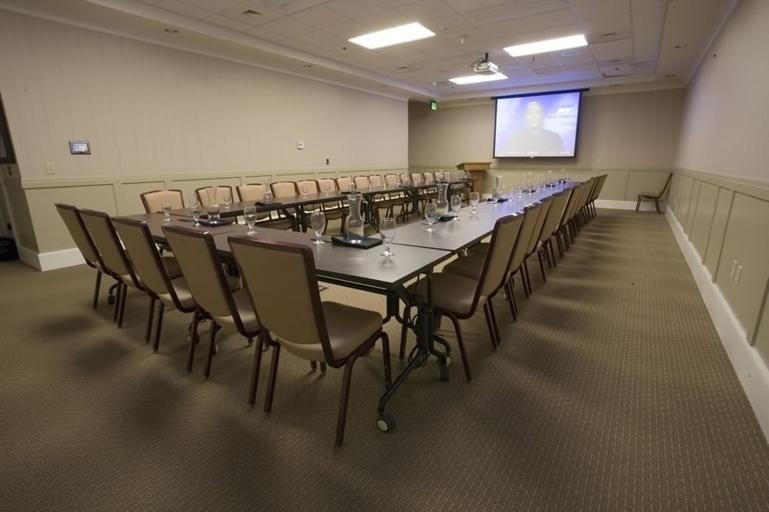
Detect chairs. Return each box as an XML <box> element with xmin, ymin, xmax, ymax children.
<box><xmin>633</xmin><ymin>171</ymin><xmax>677</xmax><ymax>216</ymax></box>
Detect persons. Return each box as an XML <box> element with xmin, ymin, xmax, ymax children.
<box><xmin>501</xmin><ymin>100</ymin><xmax>564</xmax><ymax>156</ymax></box>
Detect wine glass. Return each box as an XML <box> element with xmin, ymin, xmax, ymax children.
<box><xmin>162</xmin><ymin>168</ymin><xmax>584</xmax><ymax>258</ymax></box>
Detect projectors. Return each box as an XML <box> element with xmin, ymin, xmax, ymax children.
<box><xmin>474</xmin><ymin>62</ymin><xmax>498</xmax><ymax>74</ymax></box>
